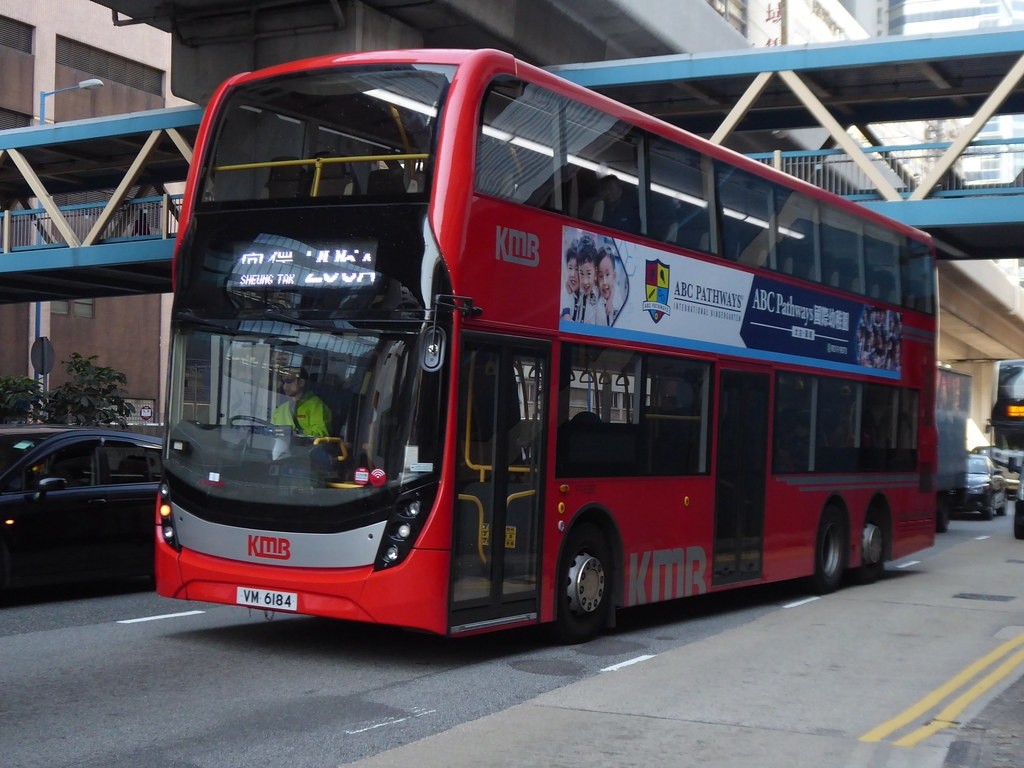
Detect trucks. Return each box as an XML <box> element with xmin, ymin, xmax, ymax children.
<box><xmin>936</xmin><ymin>407</ymin><xmax>970</xmax><ymax>534</ymax></box>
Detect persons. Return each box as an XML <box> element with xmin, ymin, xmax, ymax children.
<box><xmin>562</xmin><ymin>239</ymin><xmax>582</xmax><ymax>321</ymax></box>
<box><xmin>778</xmin><ymin>409</ymin><xmax>816</xmax><ymax>473</ymax></box>
<box><xmin>596</xmin><ymin>173</ymin><xmax>641</xmax><ymax>232</ymax></box>
<box><xmin>651</xmin><ymin>366</ymin><xmax>701</xmax><ymax>473</ymax></box>
<box><xmin>572</xmin><ymin>237</ymin><xmax>608</xmax><ymax>328</ymax></box>
<box><xmin>29</xmin><ymin>455</ymin><xmax>57</xmax><ymax>487</ymax></box>
<box><xmin>596</xmin><ymin>245</ymin><xmax>623</xmax><ymax>327</ymax></box>
<box><xmin>269</xmin><ymin>364</ymin><xmax>335</xmax><ymax>441</ymax></box>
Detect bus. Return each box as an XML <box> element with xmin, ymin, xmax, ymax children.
<box><xmin>153</xmin><ymin>45</ymin><xmax>938</xmax><ymax>637</ymax></box>
<box><xmin>983</xmin><ymin>357</ymin><xmax>1024</xmax><ymax>499</ymax></box>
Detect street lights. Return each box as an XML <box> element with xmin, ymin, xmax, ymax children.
<box><xmin>32</xmin><ymin>79</ymin><xmax>103</xmax><ymax>426</ymax></box>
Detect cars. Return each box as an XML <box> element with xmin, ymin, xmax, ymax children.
<box><xmin>1</xmin><ymin>424</ymin><xmax>167</xmax><ymax>603</ymax></box>
<box><xmin>949</xmin><ymin>451</ymin><xmax>1007</xmax><ymax>521</ymax></box>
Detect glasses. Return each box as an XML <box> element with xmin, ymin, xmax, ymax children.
<box><xmin>282</xmin><ymin>377</ymin><xmax>296</xmax><ymax>384</ymax></box>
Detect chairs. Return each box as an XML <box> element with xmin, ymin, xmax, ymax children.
<box><xmin>260</xmin><ymin>154</ymin><xmax>912</xmax><ymax>483</ymax></box>
